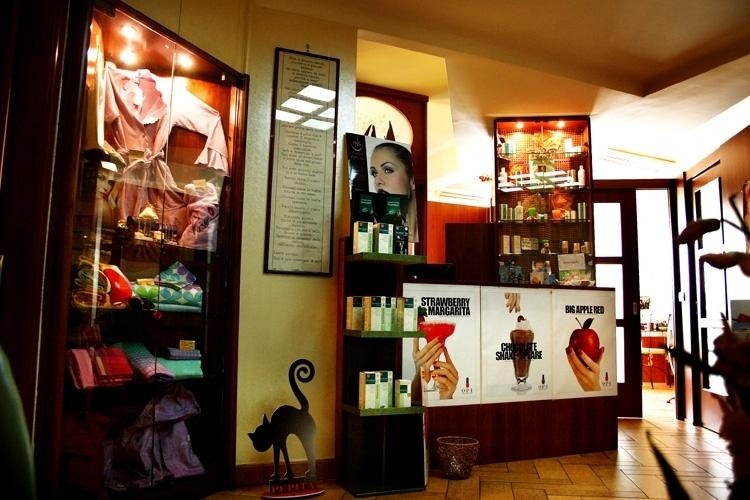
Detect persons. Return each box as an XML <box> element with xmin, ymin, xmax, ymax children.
<box><xmin>413</xmin><ymin>326</ymin><xmax>460</xmax><ymax>400</ymax></box>
<box><xmin>92</xmin><ymin>162</ymin><xmax>117</xmax><ymax>230</ymax></box>
<box><xmin>370</xmin><ymin>142</ymin><xmax>416</xmax><ymax>194</ymax></box>
<box><xmin>565</xmin><ymin>346</ymin><xmax>602</xmax><ymax>391</ymax></box>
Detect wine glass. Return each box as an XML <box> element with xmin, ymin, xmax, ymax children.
<box><xmin>510</xmin><ymin>330</ymin><xmax>534</xmax><ymax>392</ymax></box>
<box><xmin>420</xmin><ymin>321</ymin><xmax>456</xmax><ymax>392</ymax></box>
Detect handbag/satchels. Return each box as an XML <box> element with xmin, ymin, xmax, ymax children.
<box><xmin>345</xmin><ymin>122</ymin><xmax>376</xmax><ymax>166</ymax></box>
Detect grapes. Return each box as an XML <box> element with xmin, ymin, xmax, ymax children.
<box><xmin>517</xmin><ymin>315</ymin><xmax>524</xmax><ymax>323</ymax></box>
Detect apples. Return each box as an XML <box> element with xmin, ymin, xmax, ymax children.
<box><xmin>568</xmin><ymin>318</ymin><xmax>600</xmax><ymax>371</ymax></box>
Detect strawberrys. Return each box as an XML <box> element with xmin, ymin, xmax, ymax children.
<box><xmin>418</xmin><ymin>306</ymin><xmax>427</xmax><ymax>327</ymax></box>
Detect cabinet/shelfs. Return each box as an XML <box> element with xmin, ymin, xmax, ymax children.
<box><xmin>341</xmin><ymin>252</ymin><xmax>424</xmax><ymax>417</ymax></box>
<box><xmin>493</xmin><ymin>115</ymin><xmax>595</xmax><ymax>289</ymax></box>
<box><xmin>1</xmin><ymin>0</ymin><xmax>250</xmax><ymax>499</ymax></box>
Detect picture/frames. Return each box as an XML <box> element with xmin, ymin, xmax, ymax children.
<box><xmin>262</xmin><ymin>45</ymin><xmax>341</xmax><ymax>279</ymax></box>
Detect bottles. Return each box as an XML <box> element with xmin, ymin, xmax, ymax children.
<box><xmin>514</xmin><ymin>201</ymin><xmax>524</xmax><ymax>221</ymax></box>
<box><xmin>499</xmin><ymin>167</ymin><xmax>508</xmax><ymax>189</ymax></box>
<box><xmin>578</xmin><ymin>165</ymin><xmax>586</xmax><ymax>188</ymax></box>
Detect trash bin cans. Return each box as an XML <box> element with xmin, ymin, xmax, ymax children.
<box><xmin>436</xmin><ymin>436</ymin><xmax>479</xmax><ymax>480</ymax></box>
